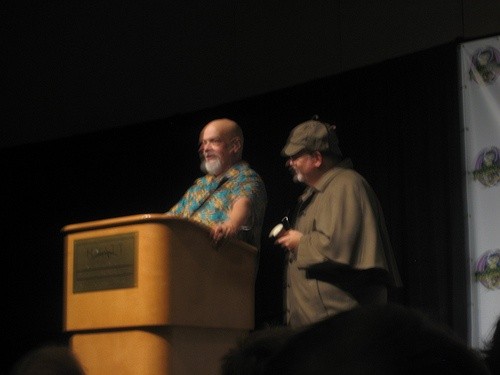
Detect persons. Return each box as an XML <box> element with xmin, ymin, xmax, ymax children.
<box><xmin>485</xmin><ymin>317</ymin><xmax>500</xmax><ymax>375</ymax></box>
<box><xmin>163</xmin><ymin>119</ymin><xmax>264</xmax><ymax>247</ymax></box>
<box><xmin>215</xmin><ymin>326</ymin><xmax>297</xmax><ymax>375</ymax></box>
<box><xmin>274</xmin><ymin>299</ymin><xmax>491</xmax><ymax>374</ymax></box>
<box><xmin>274</xmin><ymin>122</ymin><xmax>398</xmax><ymax>323</ymax></box>
<box><xmin>18</xmin><ymin>346</ymin><xmax>87</xmax><ymax>375</ymax></box>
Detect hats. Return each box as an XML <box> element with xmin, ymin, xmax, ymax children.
<box><xmin>283</xmin><ymin>120</ymin><xmax>339</xmax><ymax>156</ymax></box>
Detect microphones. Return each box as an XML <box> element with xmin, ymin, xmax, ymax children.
<box><xmin>187</xmin><ymin>175</ymin><xmax>231</xmax><ymax>218</ymax></box>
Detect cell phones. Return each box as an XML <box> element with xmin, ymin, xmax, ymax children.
<box><xmin>272</xmin><ymin>225</ymin><xmax>286</xmax><ymax>241</ymax></box>
